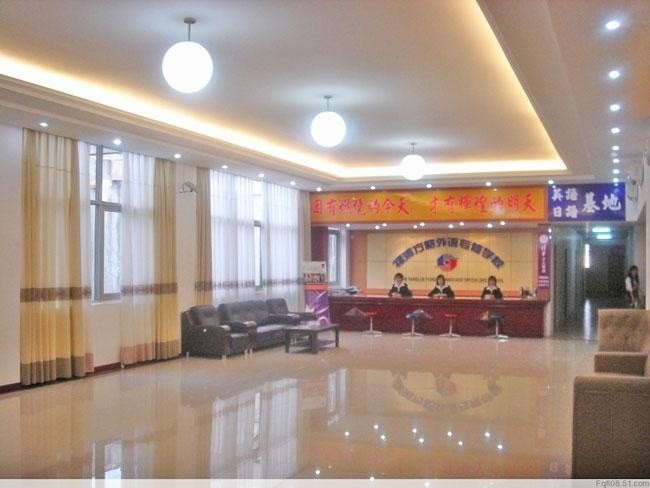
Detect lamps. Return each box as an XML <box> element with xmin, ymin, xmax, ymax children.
<box><xmin>310</xmin><ymin>94</ymin><xmax>347</xmax><ymax>147</ymax></box>
<box><xmin>161</xmin><ymin>18</ymin><xmax>213</xmax><ymax>94</ymax></box>
<box><xmin>399</xmin><ymin>143</ymin><xmax>427</xmax><ymax>181</ymax></box>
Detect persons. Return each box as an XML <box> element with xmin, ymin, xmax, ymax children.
<box><xmin>387</xmin><ymin>273</ymin><xmax>412</xmax><ymax>297</ymax></box>
<box><xmin>429</xmin><ymin>275</ymin><xmax>455</xmax><ymax>297</ymax></box>
<box><xmin>624</xmin><ymin>266</ymin><xmax>641</xmax><ymax>308</ymax></box>
<box><xmin>481</xmin><ymin>275</ymin><xmax>503</xmax><ymax>298</ymax></box>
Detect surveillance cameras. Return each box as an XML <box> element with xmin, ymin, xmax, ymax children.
<box><xmin>185</xmin><ymin>181</ymin><xmax>200</xmax><ymax>193</ymax></box>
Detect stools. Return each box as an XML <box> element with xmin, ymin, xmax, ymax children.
<box><xmin>401</xmin><ymin>313</ymin><xmax>423</xmax><ymax>339</ymax></box>
<box><xmin>440</xmin><ymin>313</ymin><xmax>461</xmax><ymax>338</ymax></box>
<box><xmin>486</xmin><ymin>316</ymin><xmax>509</xmax><ymax>340</ymax></box>
<box><xmin>364</xmin><ymin>310</ymin><xmax>382</xmax><ymax>336</ymax></box>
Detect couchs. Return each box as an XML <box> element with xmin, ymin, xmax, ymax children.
<box><xmin>218</xmin><ymin>300</ymin><xmax>294</xmax><ymax>356</ymax></box>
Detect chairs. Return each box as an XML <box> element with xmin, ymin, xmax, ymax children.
<box><xmin>266</xmin><ymin>298</ymin><xmax>314</xmax><ymax>342</ymax></box>
<box><xmin>594</xmin><ymin>308</ymin><xmax>650</xmax><ymax>375</ymax></box>
<box><xmin>572</xmin><ymin>375</ymin><xmax>650</xmax><ymax>478</ymax></box>
<box><xmin>182</xmin><ymin>303</ymin><xmax>250</xmax><ymax>360</ymax></box>
